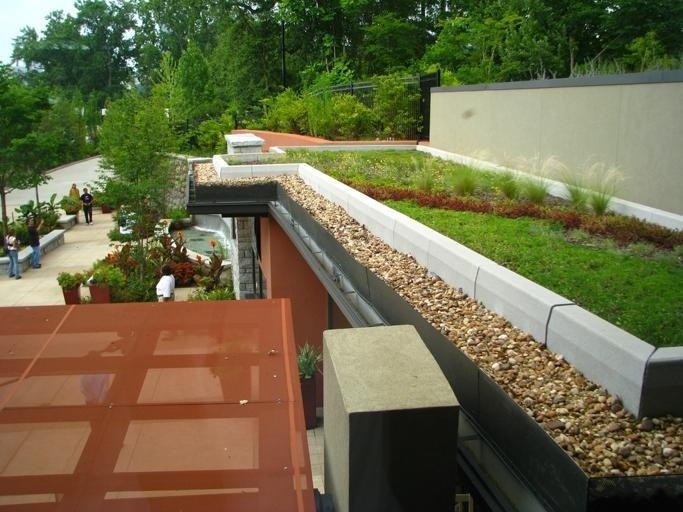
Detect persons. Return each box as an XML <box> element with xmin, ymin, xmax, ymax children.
<box><xmin>26</xmin><ymin>218</ymin><xmax>44</xmax><ymax>268</ymax></box>
<box><xmin>80</xmin><ymin>188</ymin><xmax>95</xmax><ymax>225</ymax></box>
<box><xmin>156</xmin><ymin>264</ymin><xmax>175</xmax><ymax>302</ymax></box>
<box><xmin>70</xmin><ymin>183</ymin><xmax>79</xmax><ymax>203</ymax></box>
<box><xmin>5</xmin><ymin>229</ymin><xmax>22</xmax><ymax>279</ymax></box>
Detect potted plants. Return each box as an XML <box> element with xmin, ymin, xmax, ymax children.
<box><xmin>87</xmin><ymin>176</ymin><xmax>113</xmax><ymax>213</ymax></box>
<box><xmin>56</xmin><ymin>260</ymin><xmax>127</xmax><ymax>303</ymax></box>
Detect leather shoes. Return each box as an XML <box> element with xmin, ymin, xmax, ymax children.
<box><xmin>10</xmin><ymin>274</ymin><xmax>21</xmax><ymax>279</ymax></box>
<box><xmin>33</xmin><ymin>264</ymin><xmax>41</xmax><ymax>268</ymax></box>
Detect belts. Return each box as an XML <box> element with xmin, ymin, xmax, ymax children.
<box><xmin>158</xmin><ymin>293</ymin><xmax>173</xmax><ymax>297</ymax></box>
